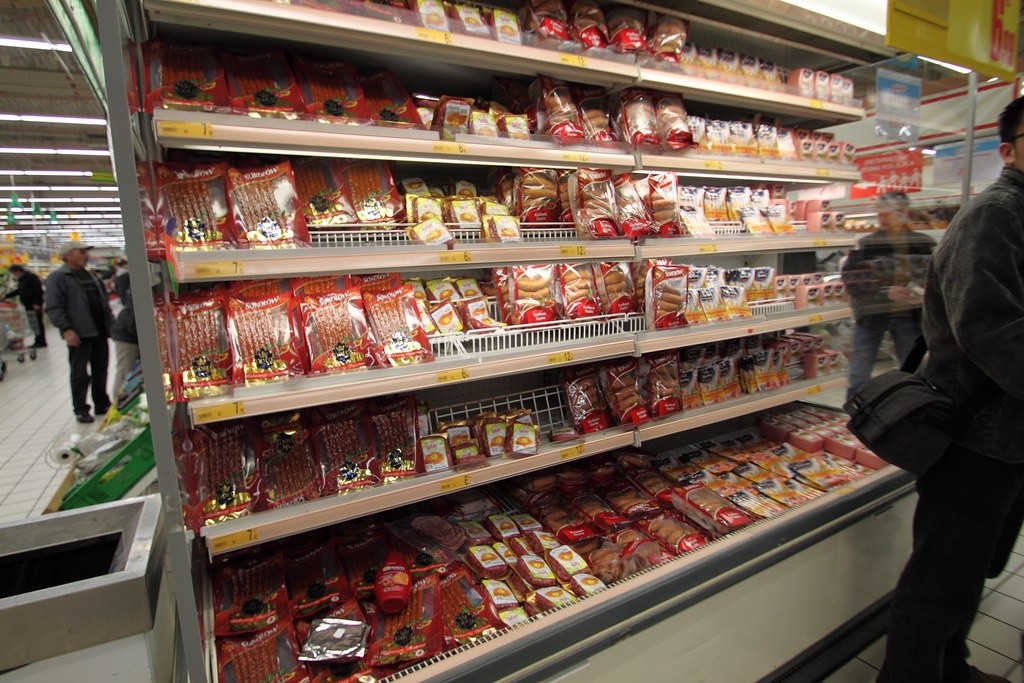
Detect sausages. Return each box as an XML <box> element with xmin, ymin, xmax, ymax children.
<box><xmin>490</xmin><ymin>0</ymin><xmax>752</xmax><ymax>585</ymax></box>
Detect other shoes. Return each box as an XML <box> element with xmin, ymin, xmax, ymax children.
<box><xmin>28</xmin><ymin>343</ymin><xmax>47</xmax><ymax>348</ymax></box>
<box><xmin>77</xmin><ymin>413</ymin><xmax>94</xmax><ymax>423</ymax></box>
<box><xmin>946</xmin><ymin>664</ymin><xmax>1013</xmax><ymax>683</ymax></box>
<box><xmin>95</xmin><ymin>406</ymin><xmax>111</xmax><ymax>414</ymax></box>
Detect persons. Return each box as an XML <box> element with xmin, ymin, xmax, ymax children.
<box><xmin>840</xmin><ymin>191</ymin><xmax>937</xmax><ymax>401</ymax></box>
<box><xmin>114</xmin><ymin>259</ymin><xmax>130</xmax><ymax>305</ymax></box>
<box><xmin>45</xmin><ymin>241</ymin><xmax>116</xmax><ymax>423</ymax></box>
<box><xmin>0</xmin><ymin>265</ymin><xmax>47</xmax><ymax>349</ymax></box>
<box><xmin>109</xmin><ymin>286</ymin><xmax>141</xmax><ymax>400</ymax></box>
<box><xmin>876</xmin><ymin>96</ymin><xmax>1024</xmax><ymax>683</ymax></box>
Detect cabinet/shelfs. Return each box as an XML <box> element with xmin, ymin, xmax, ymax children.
<box><xmin>143</xmin><ymin>0</ymin><xmax>921</xmax><ymax>683</ymax></box>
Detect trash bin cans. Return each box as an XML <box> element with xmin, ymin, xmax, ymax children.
<box><xmin>0</xmin><ymin>490</ymin><xmax>180</xmax><ymax>683</ymax></box>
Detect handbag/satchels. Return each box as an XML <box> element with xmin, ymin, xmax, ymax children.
<box><xmin>843</xmin><ymin>371</ymin><xmax>958</xmax><ymax>476</ymax></box>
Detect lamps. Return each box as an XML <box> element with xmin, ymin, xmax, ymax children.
<box><xmin>0</xmin><ymin>33</ymin><xmax>72</xmax><ymax>54</ymax></box>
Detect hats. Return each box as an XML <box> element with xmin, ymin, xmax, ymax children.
<box><xmin>59</xmin><ymin>241</ymin><xmax>96</xmax><ymax>261</ymax></box>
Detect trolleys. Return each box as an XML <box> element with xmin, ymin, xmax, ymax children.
<box><xmin>0</xmin><ymin>307</ymin><xmax>41</xmax><ymax>381</ymax></box>
